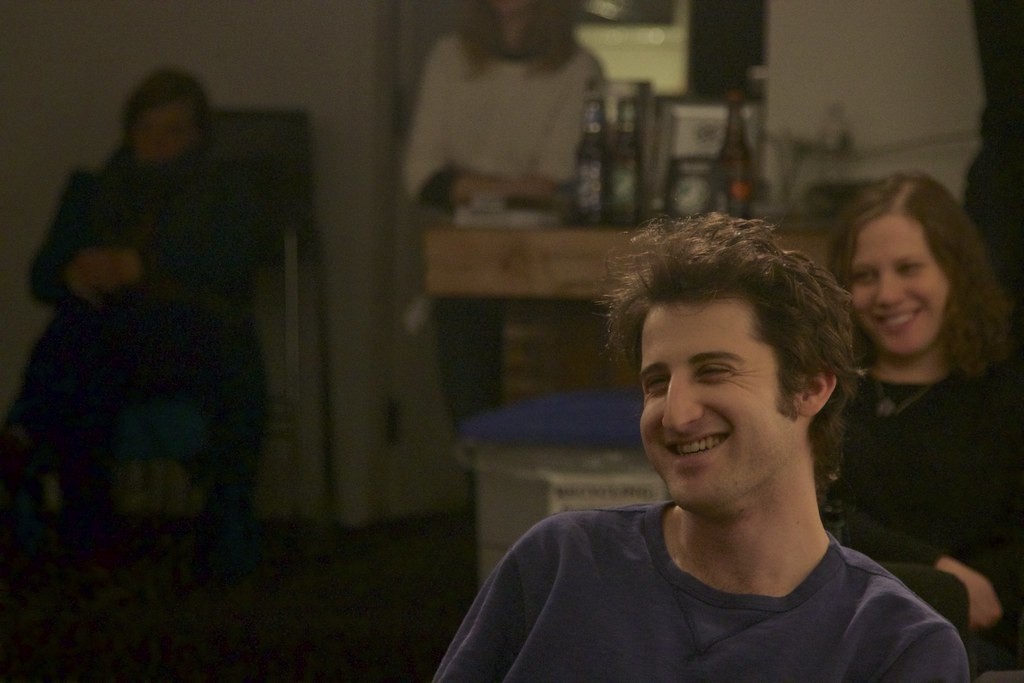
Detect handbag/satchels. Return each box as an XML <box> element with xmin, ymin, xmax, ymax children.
<box><xmin>103</xmin><ymin>392</ymin><xmax>217</xmax><ymax>518</ymax></box>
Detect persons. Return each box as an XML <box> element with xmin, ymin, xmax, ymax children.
<box><xmin>818</xmin><ymin>170</ymin><xmax>1024</xmax><ymax>683</ymax></box>
<box><xmin>402</xmin><ymin>0</ymin><xmax>610</xmax><ymax>426</ymax></box>
<box><xmin>29</xmin><ymin>66</ymin><xmax>272</xmax><ymax>624</ymax></box>
<box><xmin>431</xmin><ymin>213</ymin><xmax>972</xmax><ymax>683</ymax></box>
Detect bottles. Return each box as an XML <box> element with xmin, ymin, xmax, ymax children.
<box><xmin>570</xmin><ymin>74</ymin><xmax>860</xmax><ymax>223</ymax></box>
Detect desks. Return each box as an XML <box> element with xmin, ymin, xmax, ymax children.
<box><xmin>420</xmin><ymin>214</ymin><xmax>873</xmax><ymax>503</ymax></box>
